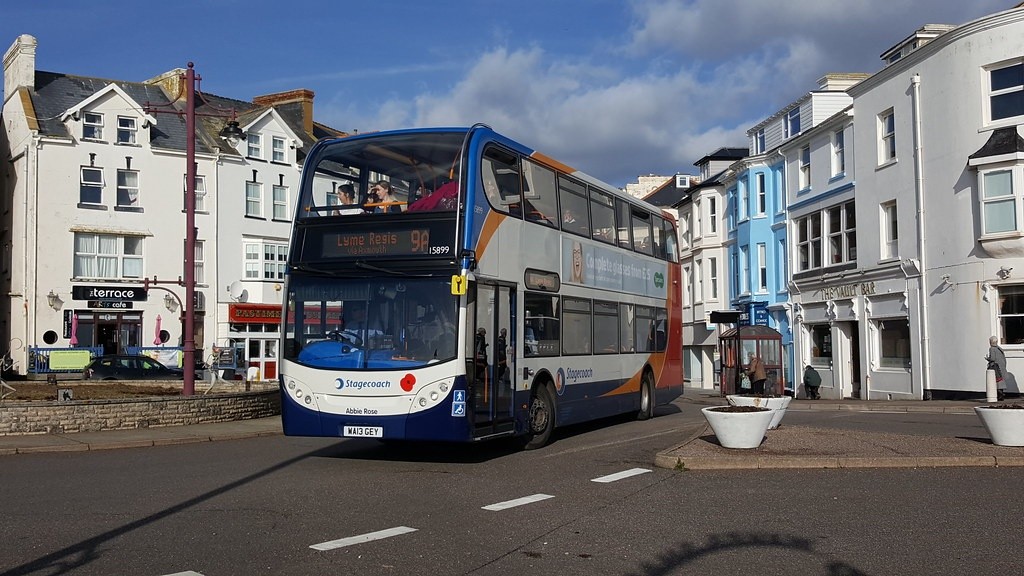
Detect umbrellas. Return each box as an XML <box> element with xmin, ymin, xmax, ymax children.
<box><xmin>69</xmin><ymin>314</ymin><xmax>79</xmax><ymax>348</ymax></box>
<box><xmin>153</xmin><ymin>314</ymin><xmax>162</xmax><ymax>347</ymax></box>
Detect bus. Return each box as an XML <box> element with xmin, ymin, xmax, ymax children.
<box><xmin>274</xmin><ymin>123</ymin><xmax>684</xmax><ymax>451</ymax></box>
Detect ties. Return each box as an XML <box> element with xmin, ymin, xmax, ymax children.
<box><xmin>355</xmin><ymin>322</ymin><xmax>363</xmax><ymax>349</ymax></box>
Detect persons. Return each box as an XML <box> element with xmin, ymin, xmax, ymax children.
<box><xmin>747</xmin><ymin>354</ymin><xmax>767</xmax><ymax>396</ymax></box>
<box><xmin>498</xmin><ymin>328</ymin><xmax>508</xmax><ymax>383</ymax></box>
<box><xmin>475</xmin><ymin>328</ymin><xmax>489</xmax><ymax>382</ymax></box>
<box><xmin>569</xmin><ymin>241</ymin><xmax>585</xmax><ymax>284</ymax></box>
<box><xmin>803</xmin><ymin>365</ymin><xmax>822</xmax><ymax>399</ymax></box>
<box><xmin>985</xmin><ymin>336</ymin><xmax>1007</xmax><ymax>401</ymax></box>
<box><xmin>332</xmin><ymin>180</ymin><xmax>402</xmax><ymax>215</ymax></box>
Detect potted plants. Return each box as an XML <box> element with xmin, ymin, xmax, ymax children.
<box><xmin>701</xmin><ymin>405</ymin><xmax>775</xmax><ymax>449</ymax></box>
<box><xmin>726</xmin><ymin>394</ymin><xmax>792</xmax><ymax>430</ymax></box>
<box><xmin>974</xmin><ymin>405</ymin><xmax>1024</xmax><ymax>446</ymax></box>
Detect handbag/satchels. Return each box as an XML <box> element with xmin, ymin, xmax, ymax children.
<box><xmin>741</xmin><ymin>373</ymin><xmax>752</xmax><ymax>389</ymax></box>
<box><xmin>987</xmin><ymin>361</ymin><xmax>1003</xmax><ymax>382</ymax></box>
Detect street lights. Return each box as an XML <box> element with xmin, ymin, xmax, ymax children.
<box><xmin>140</xmin><ymin>62</ymin><xmax>244</xmax><ymax>394</ymax></box>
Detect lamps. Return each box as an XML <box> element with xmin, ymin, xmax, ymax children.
<box><xmin>47</xmin><ymin>289</ymin><xmax>58</xmax><ymax>307</ymax></box>
<box><xmin>163</xmin><ymin>293</ymin><xmax>174</xmax><ymax>309</ymax></box>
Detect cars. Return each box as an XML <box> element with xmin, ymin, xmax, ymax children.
<box><xmin>82</xmin><ymin>351</ymin><xmax>203</xmax><ymax>381</ymax></box>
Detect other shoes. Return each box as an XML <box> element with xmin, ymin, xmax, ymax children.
<box><xmin>815</xmin><ymin>393</ymin><xmax>821</xmax><ymax>400</ymax></box>
<box><xmin>998</xmin><ymin>394</ymin><xmax>1004</xmax><ymax>401</ymax></box>
<box><xmin>805</xmin><ymin>396</ymin><xmax>811</xmax><ymax>400</ymax></box>
